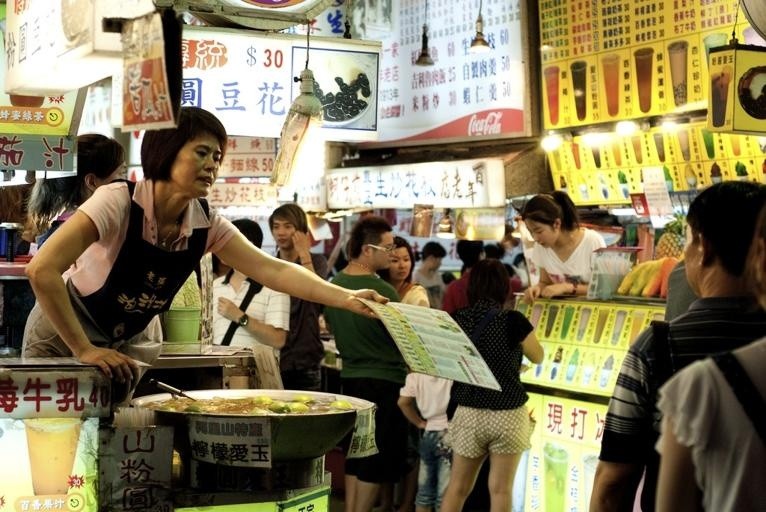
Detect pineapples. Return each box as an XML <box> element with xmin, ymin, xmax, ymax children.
<box><xmin>654</xmin><ymin>210</ymin><xmax>685</xmax><ymax>261</ymax></box>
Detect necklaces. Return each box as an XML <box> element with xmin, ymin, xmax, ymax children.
<box><xmin>154</xmin><ymin>216</ymin><xmax>180</xmax><ymax>249</ymax></box>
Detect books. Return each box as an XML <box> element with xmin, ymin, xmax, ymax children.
<box><xmin>358</xmin><ymin>296</ymin><xmax>504</xmax><ymax>393</ymax></box>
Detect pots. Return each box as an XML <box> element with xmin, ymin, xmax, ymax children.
<box><xmin>128</xmin><ymin>387</ymin><xmax>377</xmax><ymax>462</ymax></box>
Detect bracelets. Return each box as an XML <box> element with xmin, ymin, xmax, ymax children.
<box><xmin>301</xmin><ymin>260</ymin><xmax>313</xmax><ymax>268</ymax></box>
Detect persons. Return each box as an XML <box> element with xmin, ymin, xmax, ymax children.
<box><xmin>510</xmin><ymin>252</ymin><xmax>529</xmax><ymax>290</ymax></box>
<box><xmin>19</xmin><ymin>105</ymin><xmax>390</xmax><ymax>382</ymax></box>
<box><xmin>440</xmin><ymin>241</ymin><xmax>485</xmax><ymax>316</ymax></box>
<box><xmin>212</xmin><ymin>218</ymin><xmax>291</xmax><ymax>392</ymax></box>
<box><xmin>20</xmin><ymin>134</ymin><xmax>127</xmax><ymax>273</ymax></box>
<box><xmin>322</xmin><ymin>216</ymin><xmax>401</xmax><ymax>512</ymax></box>
<box><xmin>484</xmin><ymin>244</ymin><xmax>521</xmax><ymax>293</ymax></box>
<box><xmin>584</xmin><ymin>183</ymin><xmax>764</xmax><ymax>512</ymax></box>
<box><xmin>212</xmin><ymin>255</ymin><xmax>231</xmax><ymax>278</ymax></box>
<box><xmin>435</xmin><ymin>258</ymin><xmax>545</xmax><ymax>512</ymax></box>
<box><xmin>412</xmin><ymin>240</ymin><xmax>447</xmax><ymax>311</ymax></box>
<box><xmin>523</xmin><ymin>190</ymin><xmax>607</xmax><ymax>306</ymax></box>
<box><xmin>397</xmin><ymin>371</ymin><xmax>455</xmax><ymax>511</ymax></box>
<box><xmin>655</xmin><ymin>212</ymin><xmax>765</xmax><ymax>512</ymax></box>
<box><xmin>377</xmin><ymin>236</ymin><xmax>432</xmax><ymax>512</ymax></box>
<box><xmin>268</xmin><ymin>201</ymin><xmax>327</xmax><ymax>390</ymax></box>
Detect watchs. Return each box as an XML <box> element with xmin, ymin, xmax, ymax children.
<box><xmin>236</xmin><ymin>314</ymin><xmax>251</xmax><ymax>329</ymax></box>
<box><xmin>571</xmin><ymin>279</ymin><xmax>579</xmax><ymax>295</ymax></box>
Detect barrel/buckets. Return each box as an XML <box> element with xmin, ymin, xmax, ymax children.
<box><xmin>166</xmin><ymin>307</ymin><xmax>201</xmax><ymax>341</ymax></box>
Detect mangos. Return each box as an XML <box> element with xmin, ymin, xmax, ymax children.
<box><xmin>640</xmin><ymin>259</ymin><xmax>660</xmax><ymax>299</ymax></box>
<box><xmin>616</xmin><ymin>264</ymin><xmax>638</xmax><ymax>296</ymax></box>
<box><xmin>628</xmin><ymin>255</ymin><xmax>658</xmax><ymax>297</ymax></box>
<box><xmin>660</xmin><ymin>258</ymin><xmax>679</xmax><ymax>300</ymax></box>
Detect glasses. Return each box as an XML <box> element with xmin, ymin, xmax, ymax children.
<box><xmin>367</xmin><ymin>243</ymin><xmax>396</xmax><ymax>253</ymax></box>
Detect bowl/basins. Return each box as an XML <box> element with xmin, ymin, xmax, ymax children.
<box><xmin>10</xmin><ymin>95</ymin><xmax>44</xmax><ymax>107</ymax></box>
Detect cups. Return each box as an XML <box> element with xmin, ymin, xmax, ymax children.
<box><xmin>701</xmin><ymin>129</ymin><xmax>716</xmax><ymax>159</ymax></box>
<box><xmin>560</xmin><ymin>306</ymin><xmax>576</xmax><ymax>339</ymax></box>
<box><xmin>703</xmin><ymin>33</ymin><xmax>728</xmax><ymax>71</ymax></box>
<box><xmin>711</xmin><ymin>71</ymin><xmax>731</xmax><ymax>128</ymax></box>
<box><xmin>560</xmin><ymin>187</ymin><xmax>568</xmax><ymax>194</ymax></box>
<box><xmin>567</xmin><ymin>363</ymin><xmax>576</xmax><ymax>381</ymax></box>
<box><xmin>611</xmin><ymin>310</ymin><xmax>628</xmax><ymax>345</ymax></box>
<box><xmin>630</xmin><ymin>137</ymin><xmax>644</xmax><ymax>165</ymax></box>
<box><xmin>576</xmin><ymin>308</ymin><xmax>592</xmax><ymax>341</ymax></box>
<box><xmin>581</xmin><ymin>366</ymin><xmax>595</xmax><ymax>386</ymax></box>
<box><xmin>599</xmin><ymin>369</ymin><xmax>611</xmax><ymax>387</ymax></box>
<box><xmin>570</xmin><ymin>143</ymin><xmax>582</xmax><ymax>170</ymax></box>
<box><xmin>729</xmin><ymin>135</ymin><xmax>741</xmax><ymax>157</ymax></box>
<box><xmin>629</xmin><ymin>311</ymin><xmax>646</xmax><ymax>346</ymax></box>
<box><xmin>552</xmin><ymin>150</ymin><xmax>562</xmax><ymax>172</ymax></box>
<box><xmin>652</xmin><ymin>133</ymin><xmax>666</xmax><ymax>162</ymax></box>
<box><xmin>530</xmin><ymin>306</ymin><xmax>543</xmax><ymax>333</ymax></box>
<box><xmin>632</xmin><ymin>47</ymin><xmax>655</xmax><ymax>113</ymax></box>
<box><xmin>22</xmin><ymin>414</ymin><xmax>81</xmax><ymax>495</ymax></box>
<box><xmin>739</xmin><ymin>176</ymin><xmax>748</xmax><ymax>182</ymax></box>
<box><xmin>600</xmin><ymin>55</ymin><xmax>622</xmax><ymax>117</ymax></box>
<box><xmin>676</xmin><ymin>130</ymin><xmax>692</xmax><ymax>162</ymax></box>
<box><xmin>544</xmin><ymin>66</ymin><xmax>561</xmax><ymax>125</ymax></box>
<box><xmin>544</xmin><ymin>305</ymin><xmax>558</xmax><ymax>338</ymax></box>
<box><xmin>619</xmin><ymin>183</ymin><xmax>630</xmax><ymax>200</ymax></box>
<box><xmin>550</xmin><ymin>363</ymin><xmax>561</xmax><ymax>380</ymax></box>
<box><xmin>665</xmin><ymin>181</ymin><xmax>673</xmax><ymax>191</ymax></box>
<box><xmin>591</xmin><ymin>148</ymin><xmax>602</xmax><ymax>169</ymax></box>
<box><xmin>666</xmin><ymin>40</ymin><xmax>689</xmax><ymax>106</ymax></box>
<box><xmin>511</xmin><ymin>451</ymin><xmax>528</xmax><ymax>510</ymax></box>
<box><xmin>579</xmin><ymin>186</ymin><xmax>589</xmax><ymax>201</ymax></box>
<box><xmin>535</xmin><ymin>363</ymin><xmax>543</xmax><ymax>377</ymax></box>
<box><xmin>0</xmin><ymin>222</ymin><xmax>24</xmax><ymax>258</ymax></box>
<box><xmin>542</xmin><ymin>445</ymin><xmax>567</xmax><ymax>512</ymax></box>
<box><xmin>599</xmin><ymin>184</ymin><xmax>610</xmax><ymax>200</ymax></box>
<box><xmin>612</xmin><ymin>145</ymin><xmax>621</xmax><ymax>166</ymax></box>
<box><xmin>583</xmin><ymin>454</ymin><xmax>599</xmax><ymax>512</ymax></box>
<box><xmin>569</xmin><ymin>60</ymin><xmax>588</xmax><ymax>121</ymax></box>
<box><xmin>593</xmin><ymin>308</ymin><xmax>611</xmax><ymax>344</ymax></box>
<box><xmin>686</xmin><ymin>177</ymin><xmax>698</xmax><ymax>190</ymax></box>
